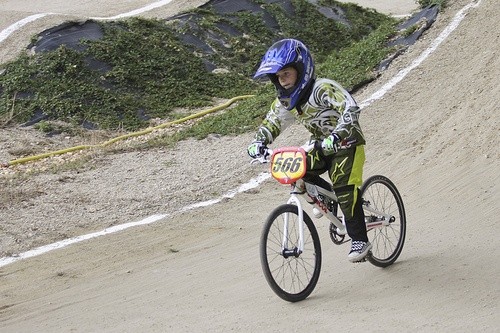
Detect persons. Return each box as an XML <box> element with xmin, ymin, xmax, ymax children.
<box><xmin>249</xmin><ymin>38</ymin><xmax>372</xmax><ymax>262</ymax></box>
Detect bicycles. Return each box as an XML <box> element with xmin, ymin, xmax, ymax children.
<box><xmin>247</xmin><ymin>140</ymin><xmax>407</xmax><ymax>302</ymax></box>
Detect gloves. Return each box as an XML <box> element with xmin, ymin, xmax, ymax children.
<box><xmin>322</xmin><ymin>132</ymin><xmax>339</xmax><ymax>156</ymax></box>
<box><xmin>248</xmin><ymin>140</ymin><xmax>262</xmax><ymax>158</ymax></box>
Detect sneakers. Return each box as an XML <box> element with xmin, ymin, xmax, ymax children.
<box><xmin>347</xmin><ymin>240</ymin><xmax>372</xmax><ymax>261</ymax></box>
<box><xmin>312</xmin><ymin>187</ymin><xmax>331</xmax><ymax>219</ymax></box>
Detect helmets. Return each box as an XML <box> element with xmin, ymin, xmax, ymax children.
<box><xmin>258</xmin><ymin>41</ymin><xmax>316</xmax><ymax>114</ymax></box>
